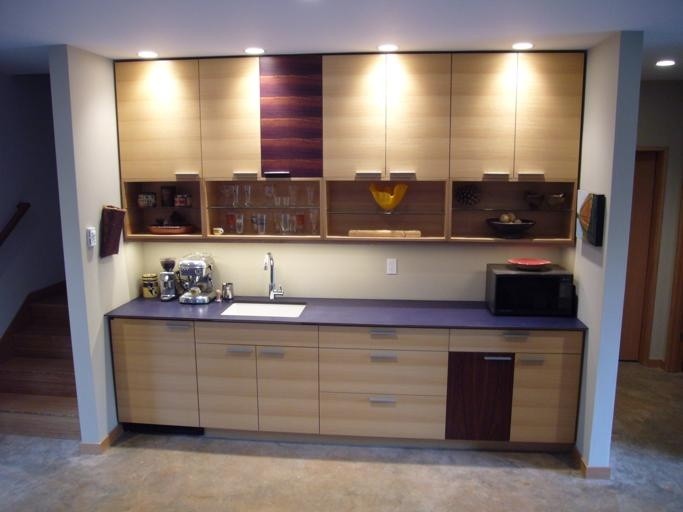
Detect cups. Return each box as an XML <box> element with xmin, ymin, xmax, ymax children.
<box><xmin>160</xmin><ymin>185</ymin><xmax>175</xmax><ymax>206</ymax></box>
<box><xmin>142</xmin><ymin>255</ymin><xmax>176</xmax><ymax>302</ymax></box>
<box><xmin>213</xmin><ymin>183</ymin><xmax>319</xmax><ymax>235</ymax></box>
<box><xmin>222</xmin><ymin>282</ymin><xmax>233</xmax><ymax>299</ymax></box>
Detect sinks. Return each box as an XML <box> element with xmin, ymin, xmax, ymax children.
<box><xmin>221</xmin><ymin>302</ymin><xmax>307</xmax><ymax>318</ymax></box>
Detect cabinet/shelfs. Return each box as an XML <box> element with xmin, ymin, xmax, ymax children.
<box><xmin>446</xmin><ymin>328</ymin><xmax>585</xmax><ymax>451</ymax></box>
<box><xmin>199</xmin><ymin>56</ymin><xmax>323</xmax><ymax>177</ymax></box>
<box><xmin>193</xmin><ymin>321</ymin><xmax>319</xmax><ymax>444</ymax></box>
<box><xmin>113</xmin><ymin>59</ymin><xmax>202</xmax><ymax>181</ymax></box>
<box><xmin>317</xmin><ymin>326</ymin><xmax>448</xmax><ymax>450</ymax></box>
<box><xmin>106</xmin><ymin>316</ymin><xmax>199</xmax><ymax>428</ymax></box>
<box><xmin>119</xmin><ymin>179</ymin><xmax>576</xmax><ymax>245</ymax></box>
<box><xmin>321</xmin><ymin>55</ymin><xmax>451</xmax><ymax>179</ymax></box>
<box><xmin>450</xmin><ymin>53</ymin><xmax>583</xmax><ymax>178</ymax></box>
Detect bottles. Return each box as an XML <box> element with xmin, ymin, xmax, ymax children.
<box><xmin>523</xmin><ymin>190</ymin><xmax>567</xmax><ymax>210</ymax></box>
<box><xmin>174</xmin><ymin>191</ymin><xmax>193</xmax><ymax>207</ymax></box>
<box><xmin>138</xmin><ymin>193</ymin><xmax>155</xmax><ymax>208</ymax></box>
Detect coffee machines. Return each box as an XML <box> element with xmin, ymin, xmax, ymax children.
<box><xmin>179</xmin><ymin>252</ymin><xmax>217</xmax><ymax>304</ymax></box>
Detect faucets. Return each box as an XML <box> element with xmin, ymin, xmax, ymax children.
<box><xmin>262</xmin><ymin>251</ymin><xmax>283</xmax><ymax>302</ymax></box>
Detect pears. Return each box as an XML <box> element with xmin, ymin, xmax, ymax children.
<box><xmin>500</xmin><ymin>213</ymin><xmax>522</xmax><ymax>223</ymax></box>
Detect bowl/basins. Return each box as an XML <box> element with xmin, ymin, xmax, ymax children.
<box><xmin>487</xmin><ymin>217</ymin><xmax>535</xmax><ymax>238</ymax></box>
<box><xmin>368</xmin><ymin>183</ymin><xmax>408</xmax><ymax>214</ymax></box>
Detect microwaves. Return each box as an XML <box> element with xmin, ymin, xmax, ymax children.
<box><xmin>485</xmin><ymin>262</ymin><xmax>572</xmax><ymax>317</ymax></box>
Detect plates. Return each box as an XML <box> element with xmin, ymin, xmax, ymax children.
<box><xmin>505</xmin><ymin>258</ymin><xmax>553</xmax><ymax>271</ymax></box>
